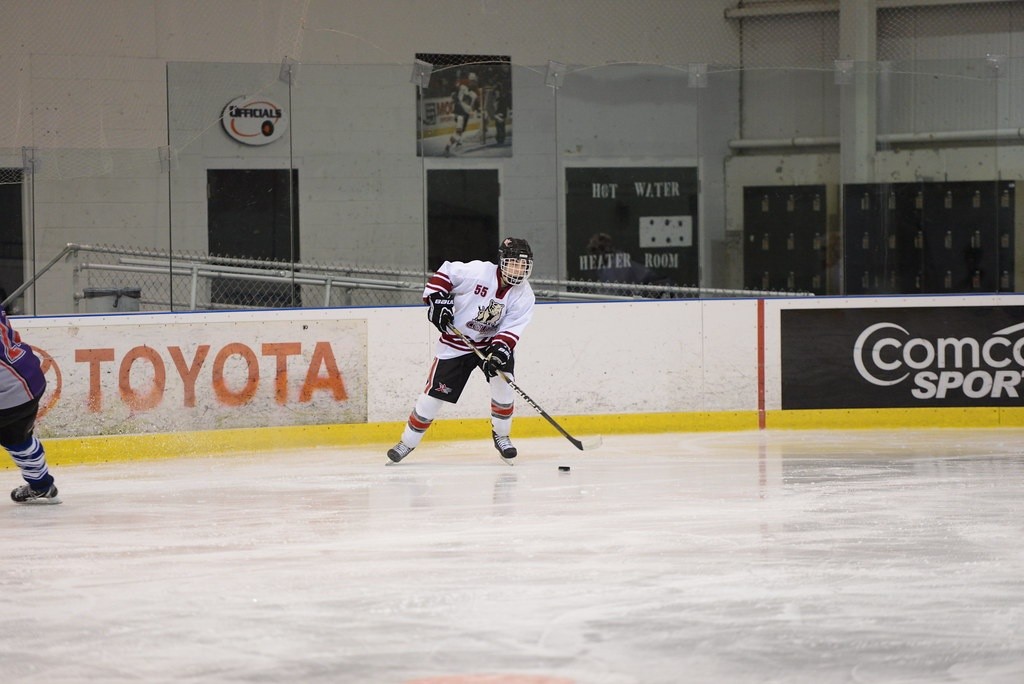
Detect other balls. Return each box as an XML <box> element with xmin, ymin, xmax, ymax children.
<box><xmin>559</xmin><ymin>464</ymin><xmax>571</xmax><ymax>472</ymax></box>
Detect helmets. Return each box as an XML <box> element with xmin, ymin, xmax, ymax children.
<box><xmin>498</xmin><ymin>237</ymin><xmax>534</xmax><ymax>261</ymax></box>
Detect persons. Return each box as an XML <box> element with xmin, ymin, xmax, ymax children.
<box><xmin>0</xmin><ymin>291</ymin><xmax>62</xmax><ymax>507</ymax></box>
<box><xmin>385</xmin><ymin>236</ymin><xmax>536</xmax><ymax>467</ymax></box>
<box><xmin>443</xmin><ymin>73</ymin><xmax>481</xmax><ymax>158</ymax></box>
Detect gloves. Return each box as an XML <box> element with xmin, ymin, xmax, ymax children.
<box><xmin>428</xmin><ymin>290</ymin><xmax>454</xmax><ymax>333</ymax></box>
<box><xmin>483</xmin><ymin>344</ymin><xmax>511</xmax><ymax>377</ymax></box>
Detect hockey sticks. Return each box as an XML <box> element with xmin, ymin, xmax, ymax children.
<box><xmin>445</xmin><ymin>320</ymin><xmax>604</xmax><ymax>454</ymax></box>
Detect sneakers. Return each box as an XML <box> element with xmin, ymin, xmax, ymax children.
<box><xmin>492</xmin><ymin>430</ymin><xmax>517</xmax><ymax>466</ymax></box>
<box><xmin>11</xmin><ymin>483</ymin><xmax>64</xmax><ymax>504</ymax></box>
<box><xmin>384</xmin><ymin>440</ymin><xmax>416</xmax><ymax>466</ymax></box>
